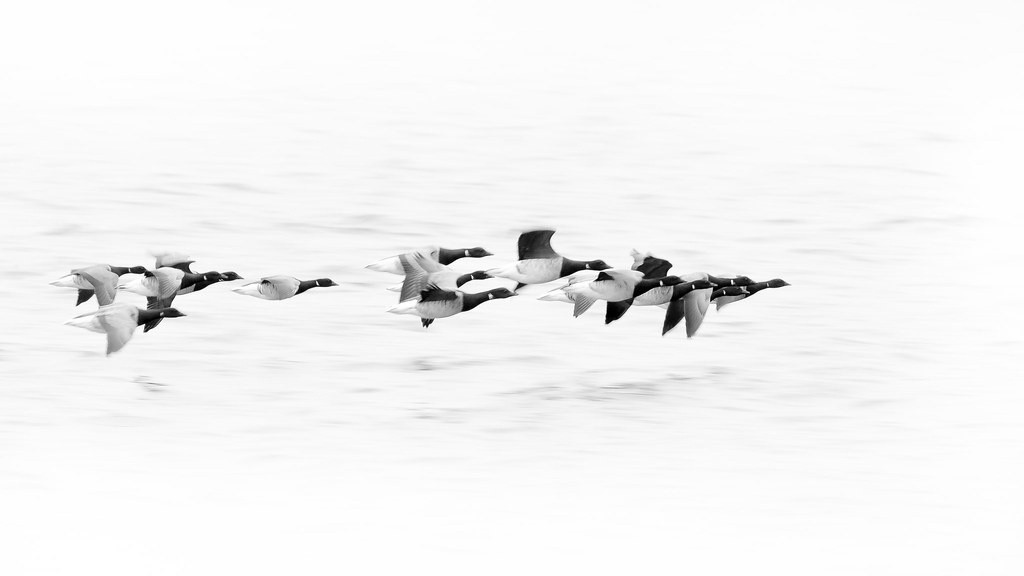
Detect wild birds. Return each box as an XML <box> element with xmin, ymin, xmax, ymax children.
<box><xmin>49</xmin><ymin>251</ymin><xmax>341</xmax><ymax>356</ymax></box>
<box><xmin>363</xmin><ymin>226</ymin><xmax>792</xmax><ymax>340</ymax></box>
